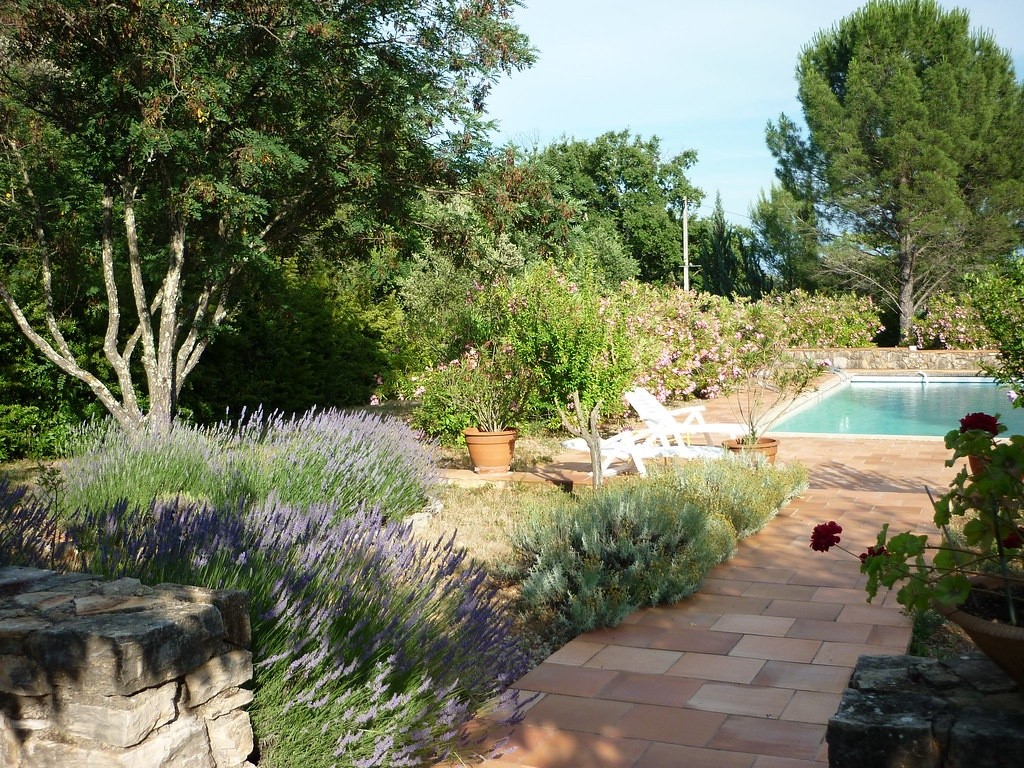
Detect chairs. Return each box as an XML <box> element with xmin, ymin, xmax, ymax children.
<box><xmin>626</xmin><ymin>386</ymin><xmax>761</xmax><ymax>447</ymax></box>
<box><xmin>558</xmin><ymin>431</ymin><xmax>724</xmax><ymax>478</ymax></box>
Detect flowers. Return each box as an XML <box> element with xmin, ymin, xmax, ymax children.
<box><xmin>703</xmin><ymin>336</ymin><xmax>830</xmax><ymax>442</ymax></box>
<box><xmin>411</xmin><ymin>349</ymin><xmax>520</xmax><ymax>432</ymax></box>
<box><xmin>810</xmin><ymin>411</ymin><xmax>1024</xmax><ymax>627</ymax></box>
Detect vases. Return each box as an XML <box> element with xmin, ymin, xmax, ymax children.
<box><xmin>933</xmin><ymin>573</ymin><xmax>1024</xmax><ymax>689</ymax></box>
<box><xmin>723</xmin><ymin>437</ymin><xmax>780</xmax><ymax>465</ymax></box>
<box><xmin>463</xmin><ymin>427</ymin><xmax>519</xmax><ymax>477</ymax></box>
